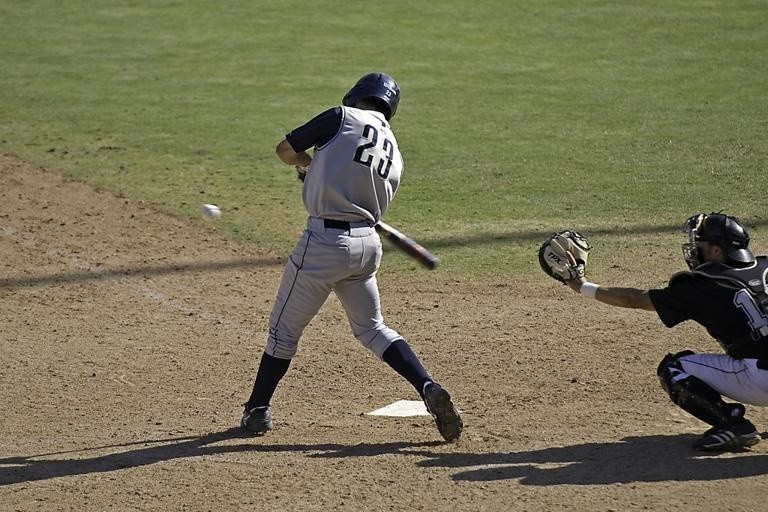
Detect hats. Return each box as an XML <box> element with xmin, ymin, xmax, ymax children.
<box><xmin>703</xmin><ymin>213</ymin><xmax>757</xmax><ymax>263</ymax></box>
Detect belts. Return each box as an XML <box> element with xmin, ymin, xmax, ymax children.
<box><xmin>324</xmin><ymin>219</ymin><xmax>350</xmax><ymax>231</ymax></box>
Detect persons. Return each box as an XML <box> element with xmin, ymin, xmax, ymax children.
<box><xmin>539</xmin><ymin>214</ymin><xmax>768</xmax><ymax>455</ymax></box>
<box><xmin>241</xmin><ymin>71</ymin><xmax>463</xmax><ymax>443</ymax></box>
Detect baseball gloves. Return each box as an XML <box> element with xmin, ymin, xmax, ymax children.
<box><xmin>539</xmin><ymin>228</ymin><xmax>591</xmax><ymax>282</ymax></box>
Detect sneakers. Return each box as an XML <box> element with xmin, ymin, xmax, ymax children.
<box><xmin>241</xmin><ymin>406</ymin><xmax>275</xmax><ymax>437</ymax></box>
<box><xmin>424</xmin><ymin>383</ymin><xmax>463</xmax><ymax>441</ymax></box>
<box><xmin>694</xmin><ymin>420</ymin><xmax>761</xmax><ymax>456</ymax></box>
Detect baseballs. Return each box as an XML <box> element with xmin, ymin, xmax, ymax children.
<box><xmin>202</xmin><ymin>204</ymin><xmax>220</xmax><ymax>221</ymax></box>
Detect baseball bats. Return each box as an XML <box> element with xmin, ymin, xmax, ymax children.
<box><xmin>374</xmin><ymin>221</ymin><xmax>441</xmax><ymax>271</ymax></box>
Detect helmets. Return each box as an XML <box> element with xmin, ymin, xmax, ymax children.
<box><xmin>342</xmin><ymin>72</ymin><xmax>401</xmax><ymax>120</ymax></box>
